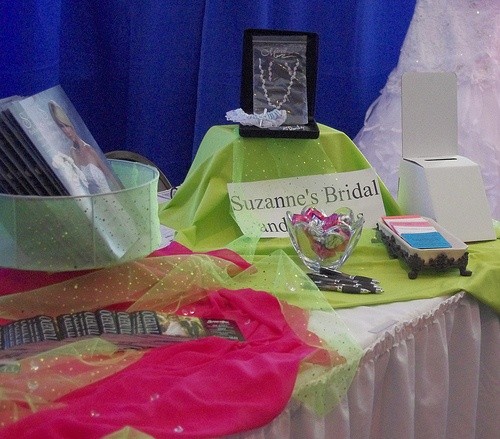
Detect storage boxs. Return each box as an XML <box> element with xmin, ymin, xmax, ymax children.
<box><xmin>398</xmin><ymin>72</ymin><xmax>496</xmax><ymax>241</ymax></box>
<box><xmin>285</xmin><ymin>204</ymin><xmax>365</xmax><ymax>272</ymax></box>
<box><xmin>378</xmin><ymin>215</ymin><xmax>472</xmax><ymax>280</ymax></box>
<box><xmin>0</xmin><ymin>159</ymin><xmax>161</xmax><ymax>272</ymax></box>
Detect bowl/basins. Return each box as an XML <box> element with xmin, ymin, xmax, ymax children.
<box><xmin>284</xmin><ymin>203</ymin><xmax>364</xmax><ymax>271</ymax></box>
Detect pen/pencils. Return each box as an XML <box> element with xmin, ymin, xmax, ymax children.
<box><xmin>302</xmin><ymin>262</ymin><xmax>386</xmax><ymax>296</ymax></box>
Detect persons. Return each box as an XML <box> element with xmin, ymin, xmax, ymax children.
<box><xmin>47</xmin><ymin>98</ymin><xmax>121</xmax><ymax>194</ymax></box>
<box><xmin>166</xmin><ymin>315</ymin><xmax>202</xmax><ymax>336</ymax></box>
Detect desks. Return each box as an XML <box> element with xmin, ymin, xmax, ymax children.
<box><xmin>1</xmin><ymin>186</ymin><xmax>500</xmax><ymax>439</ymax></box>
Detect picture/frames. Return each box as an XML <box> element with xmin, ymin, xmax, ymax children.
<box><xmin>240</xmin><ymin>29</ymin><xmax>319</xmax><ymax>139</ymax></box>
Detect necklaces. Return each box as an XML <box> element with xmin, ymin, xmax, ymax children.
<box><xmin>257</xmin><ymin>57</ymin><xmax>301</xmax><ymax>110</ymax></box>
